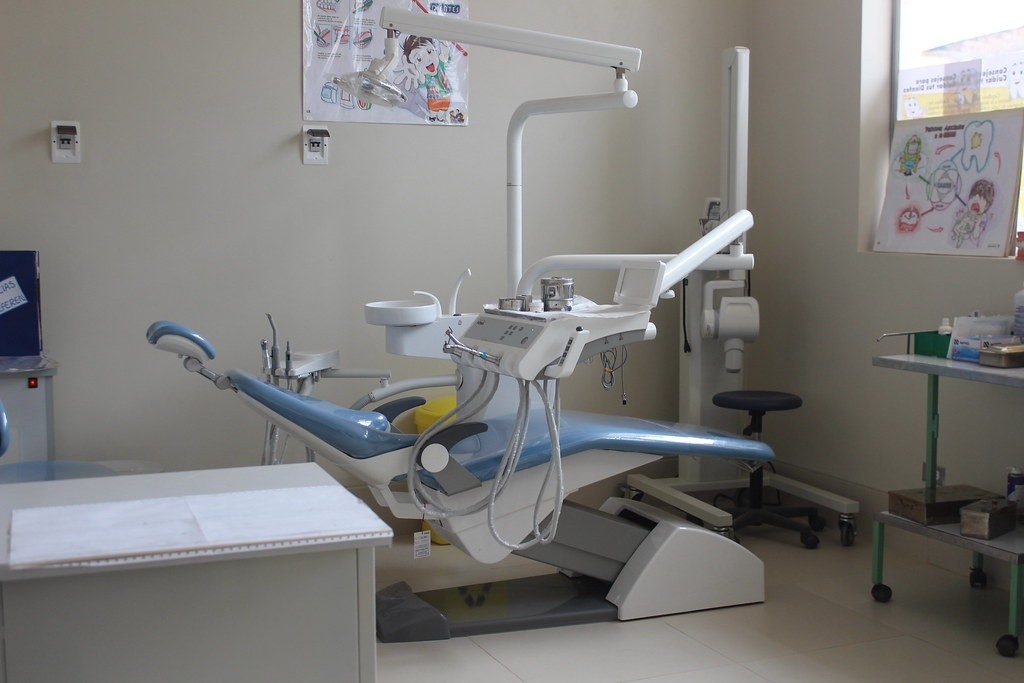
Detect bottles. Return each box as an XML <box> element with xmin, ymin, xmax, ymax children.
<box><xmin>938</xmin><ymin>318</ymin><xmax>952</xmax><ymax>336</ymax></box>
<box><xmin>1014</xmin><ymin>289</ymin><xmax>1024</xmax><ymax>339</ymax></box>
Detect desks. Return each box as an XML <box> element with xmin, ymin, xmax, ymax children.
<box><xmin>0</xmin><ymin>461</ymin><xmax>392</xmax><ymax>683</ymax></box>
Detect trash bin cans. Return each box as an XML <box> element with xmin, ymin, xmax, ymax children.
<box><xmin>413</xmin><ymin>395</ymin><xmax>456</xmax><ymax>546</ymax></box>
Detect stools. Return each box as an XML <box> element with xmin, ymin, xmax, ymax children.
<box><xmin>712</xmin><ymin>389</ymin><xmax>826</xmax><ymax>549</ymax></box>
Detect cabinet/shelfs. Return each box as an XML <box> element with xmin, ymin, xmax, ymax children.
<box><xmin>872</xmin><ymin>357</ymin><xmax>1024</xmax><ymax>658</ymax></box>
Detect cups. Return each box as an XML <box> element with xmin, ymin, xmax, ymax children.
<box><xmin>541</xmin><ymin>278</ymin><xmax>575</xmax><ymax>310</ymax></box>
<box><xmin>497</xmin><ymin>298</ymin><xmax>523</xmax><ymax>311</ymax></box>
<box><xmin>516</xmin><ymin>294</ymin><xmax>532</xmax><ymax>311</ymax></box>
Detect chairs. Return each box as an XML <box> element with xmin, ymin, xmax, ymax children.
<box><xmin>145</xmin><ymin>318</ymin><xmax>774</xmax><ymax>487</ymax></box>
